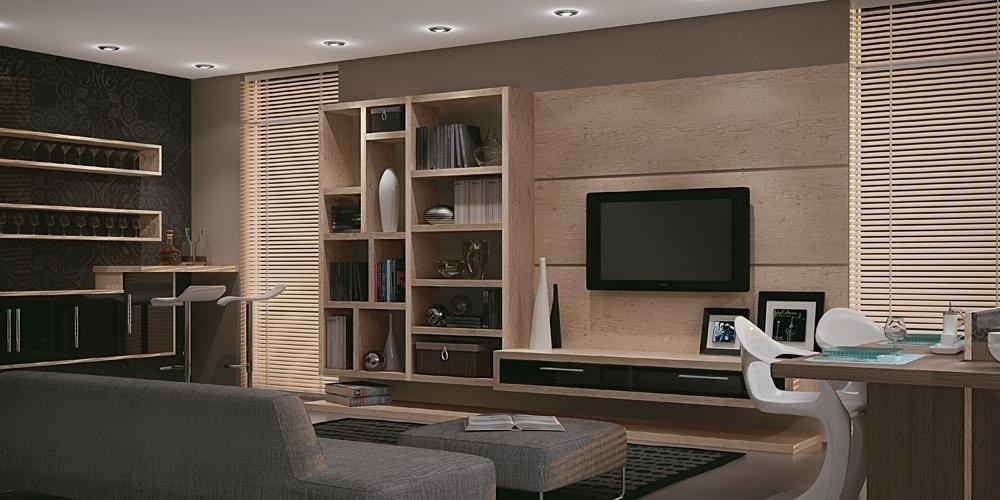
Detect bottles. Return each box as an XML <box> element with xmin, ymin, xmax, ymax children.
<box><xmin>181</xmin><ymin>227</ymin><xmax>194</xmax><ymax>265</ymax></box>
<box><xmin>193</xmin><ymin>227</ymin><xmax>208</xmax><ymax>266</ymax></box>
<box><xmin>159</xmin><ymin>230</ymin><xmax>182</xmax><ymax>265</ymax></box>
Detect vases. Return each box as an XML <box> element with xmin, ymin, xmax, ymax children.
<box><xmin>378</xmin><ymin>167</ymin><xmax>402</xmax><ymax>232</ymax></box>
<box><xmin>530</xmin><ymin>258</ymin><xmax>562</xmax><ymax>352</ymax></box>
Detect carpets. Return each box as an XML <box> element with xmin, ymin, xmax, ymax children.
<box><xmin>312</xmin><ymin>412</ymin><xmax>746</xmax><ymax>500</ymax></box>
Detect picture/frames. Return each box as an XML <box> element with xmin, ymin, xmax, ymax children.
<box><xmin>758</xmin><ymin>289</ymin><xmax>826</xmax><ymax>353</ymax></box>
<box><xmin>700</xmin><ymin>306</ymin><xmax>751</xmax><ymax>352</ymax></box>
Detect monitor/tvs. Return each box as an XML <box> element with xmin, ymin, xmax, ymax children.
<box><xmin>586</xmin><ymin>186</ymin><xmax>750</xmax><ymax>290</ymax></box>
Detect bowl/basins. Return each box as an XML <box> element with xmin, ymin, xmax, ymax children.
<box><xmin>432</xmin><ymin>259</ymin><xmax>466</xmax><ymax>278</ymax></box>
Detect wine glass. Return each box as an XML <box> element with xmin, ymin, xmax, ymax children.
<box><xmin>0</xmin><ymin>212</ymin><xmax>154</xmax><ymax>238</ymax></box>
<box><xmin>0</xmin><ymin>136</ymin><xmax>156</xmax><ymax>169</ymax></box>
<box><xmin>885</xmin><ymin>317</ymin><xmax>907</xmax><ymax>357</ymax></box>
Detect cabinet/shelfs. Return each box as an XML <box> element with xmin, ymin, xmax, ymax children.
<box><xmin>0</xmin><ymin>290</ymin><xmax>55</xmax><ymax>368</ymax></box>
<box><xmin>123</xmin><ymin>272</ymin><xmax>178</xmax><ymax>356</ymax></box>
<box><xmin>0</xmin><ymin>127</ymin><xmax>163</xmax><ymax>242</ymax></box>
<box><xmin>304</xmin><ymin>345</ymin><xmax>829</xmax><ymax>456</ymax></box>
<box><xmin>317</xmin><ymin>85</ymin><xmax>535</xmax><ymax>386</ymax></box>
<box><xmin>55</xmin><ymin>290</ymin><xmax>121</xmax><ymax>362</ymax></box>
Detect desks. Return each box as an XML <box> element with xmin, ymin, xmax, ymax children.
<box><xmin>772</xmin><ymin>331</ymin><xmax>1000</xmax><ymax>500</ymax></box>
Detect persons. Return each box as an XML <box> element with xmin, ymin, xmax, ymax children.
<box><xmin>717</xmin><ymin>321</ymin><xmax>735</xmax><ymax>342</ymax></box>
<box><xmin>776</xmin><ymin>312</ymin><xmax>807</xmax><ymax>341</ymax></box>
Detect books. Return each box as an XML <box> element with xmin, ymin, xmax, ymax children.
<box><xmin>323</xmin><ymin>391</ymin><xmax>393</xmax><ymax>409</ymax></box>
<box><xmin>442</xmin><ymin>285</ymin><xmax>504</xmax><ymax>330</ymax></box>
<box><xmin>463</xmin><ymin>413</ymin><xmax>566</xmax><ymax>432</ymax></box>
<box><xmin>414</xmin><ymin>120</ymin><xmax>482</xmax><ymax>171</ymax></box>
<box><xmin>323</xmin><ymin>313</ymin><xmax>354</xmax><ymax>370</ymax></box>
<box><xmin>324</xmin><ymin>381</ymin><xmax>396</xmax><ymax>397</ymax></box>
<box><xmin>324</xmin><ymin>204</ymin><xmax>408</xmax><ymax>304</ymax></box>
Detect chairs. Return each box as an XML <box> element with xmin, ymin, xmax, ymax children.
<box><xmin>733</xmin><ymin>308</ymin><xmax>891</xmax><ymax>500</ymax></box>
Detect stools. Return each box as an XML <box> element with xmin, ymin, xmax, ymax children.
<box><xmin>151</xmin><ymin>283</ymin><xmax>287</xmax><ymax>382</ymax></box>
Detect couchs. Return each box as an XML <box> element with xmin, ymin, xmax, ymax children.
<box><xmin>0</xmin><ymin>368</ymin><xmax>497</xmax><ymax>500</ymax></box>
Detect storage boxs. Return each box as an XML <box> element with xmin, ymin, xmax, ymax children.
<box><xmin>369</xmin><ymin>106</ymin><xmax>405</xmax><ymax>132</ymax></box>
<box><xmin>416</xmin><ymin>342</ymin><xmax>490</xmax><ymax>377</ymax></box>
